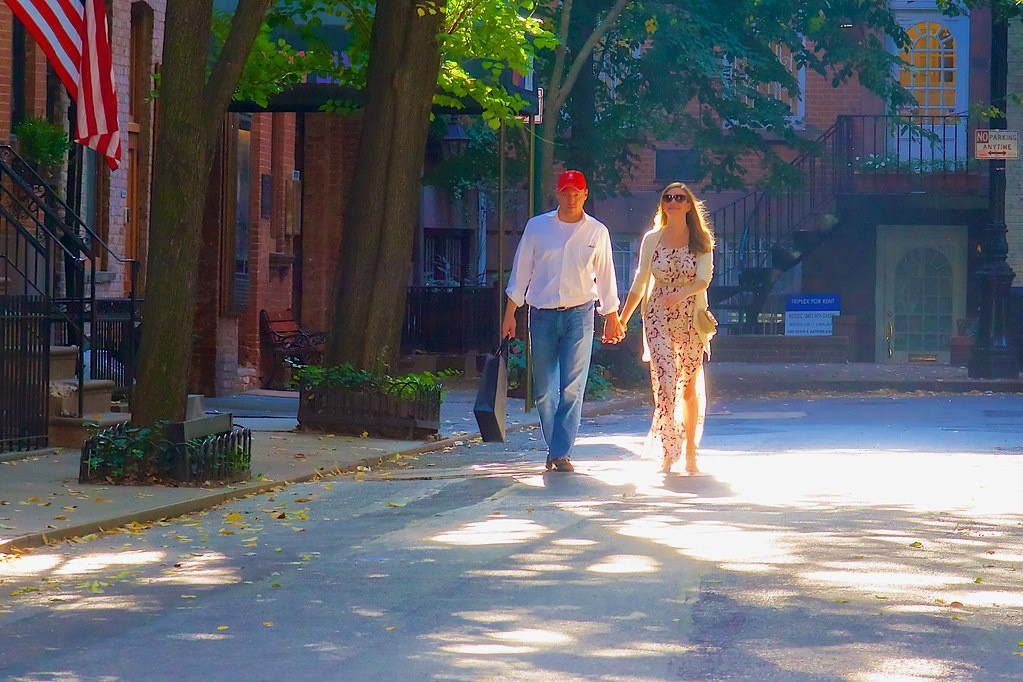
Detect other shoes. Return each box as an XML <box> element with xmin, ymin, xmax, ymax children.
<box><xmin>662</xmin><ymin>458</ymin><xmax>670</xmax><ymax>474</ymax></box>
<box><xmin>685</xmin><ymin>447</ymin><xmax>699</xmax><ymax>472</ymax></box>
<box><xmin>546</xmin><ymin>455</ymin><xmax>574</xmax><ymax>472</ymax></box>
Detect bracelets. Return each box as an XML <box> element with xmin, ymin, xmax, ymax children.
<box><xmin>620</xmin><ymin>319</ymin><xmax>628</xmax><ymax>331</ymax></box>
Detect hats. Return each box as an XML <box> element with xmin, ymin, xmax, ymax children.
<box><xmin>558</xmin><ymin>170</ymin><xmax>587</xmax><ymax>193</ymax></box>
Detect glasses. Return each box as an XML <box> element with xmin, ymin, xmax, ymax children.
<box><xmin>662</xmin><ymin>194</ymin><xmax>686</xmax><ymax>203</ymax></box>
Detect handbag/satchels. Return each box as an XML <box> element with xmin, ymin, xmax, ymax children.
<box><xmin>698</xmin><ymin>311</ymin><xmax>718</xmax><ymax>333</ymax></box>
<box><xmin>473</xmin><ymin>335</ymin><xmax>506</xmax><ymax>443</ymax></box>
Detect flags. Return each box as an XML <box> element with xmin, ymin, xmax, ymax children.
<box><xmin>5</xmin><ymin>0</ymin><xmax>122</xmax><ymax>171</ymax></box>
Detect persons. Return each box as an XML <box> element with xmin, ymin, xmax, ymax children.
<box><xmin>603</xmin><ymin>182</ymin><xmax>714</xmax><ymax>474</ymax></box>
<box><xmin>501</xmin><ymin>171</ymin><xmax>625</xmax><ymax>472</ymax></box>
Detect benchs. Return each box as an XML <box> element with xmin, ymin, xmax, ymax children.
<box><xmin>260</xmin><ymin>309</ymin><xmax>325</xmax><ymax>383</ymax></box>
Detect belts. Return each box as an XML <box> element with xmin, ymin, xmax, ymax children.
<box><xmin>540</xmin><ymin>305</ymin><xmax>582</xmax><ymax>311</ymax></box>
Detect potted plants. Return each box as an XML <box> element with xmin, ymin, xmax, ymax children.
<box><xmin>15</xmin><ymin>115</ymin><xmax>74</xmax><ymax>186</ymax></box>
<box><xmin>849</xmin><ymin>152</ymin><xmax>980</xmax><ymax>193</ymax></box>
